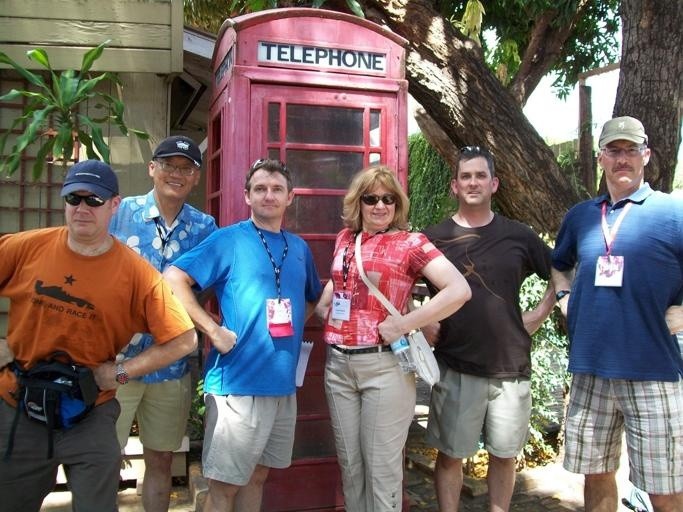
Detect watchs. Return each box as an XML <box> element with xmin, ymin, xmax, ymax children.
<box><xmin>116</xmin><ymin>362</ymin><xmax>129</xmax><ymax>385</ymax></box>
<box><xmin>557</xmin><ymin>290</ymin><xmax>569</xmax><ymax>300</ymax></box>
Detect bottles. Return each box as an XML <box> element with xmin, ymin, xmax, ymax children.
<box><xmin>381</xmin><ymin>313</ymin><xmax>417</xmax><ymax>374</ymax></box>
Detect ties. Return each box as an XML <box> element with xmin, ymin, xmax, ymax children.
<box><xmin>331</xmin><ymin>343</ymin><xmax>391</xmax><ymax>354</ymax></box>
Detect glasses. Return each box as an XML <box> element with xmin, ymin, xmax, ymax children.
<box><xmin>360</xmin><ymin>193</ymin><xmax>396</xmax><ymax>204</ymax></box>
<box><xmin>65</xmin><ymin>193</ymin><xmax>105</xmax><ymax>206</ymax></box>
<box><xmin>156</xmin><ymin>159</ymin><xmax>195</xmax><ymax>175</ymax></box>
<box><xmin>459</xmin><ymin>145</ymin><xmax>488</xmax><ymax>156</ymax></box>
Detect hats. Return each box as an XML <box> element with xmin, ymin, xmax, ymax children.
<box><xmin>153</xmin><ymin>136</ymin><xmax>202</xmax><ymax>168</ymax></box>
<box><xmin>61</xmin><ymin>159</ymin><xmax>118</xmax><ymax>200</ymax></box>
<box><xmin>599</xmin><ymin>116</ymin><xmax>649</xmax><ymax>147</ymax></box>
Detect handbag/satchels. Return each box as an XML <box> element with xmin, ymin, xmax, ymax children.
<box><xmin>9</xmin><ymin>351</ymin><xmax>98</xmax><ymax>429</ymax></box>
<box><xmin>407</xmin><ymin>331</ymin><xmax>440</xmax><ymax>385</ymax></box>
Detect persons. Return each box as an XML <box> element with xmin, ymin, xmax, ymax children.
<box><xmin>0</xmin><ymin>159</ymin><xmax>198</xmax><ymax>512</ymax></box>
<box><xmin>106</xmin><ymin>135</ymin><xmax>218</xmax><ymax>512</ymax></box>
<box><xmin>314</xmin><ymin>167</ymin><xmax>472</xmax><ymax>512</ymax></box>
<box><xmin>417</xmin><ymin>146</ymin><xmax>557</xmax><ymax>512</ymax></box>
<box><xmin>551</xmin><ymin>116</ymin><xmax>683</xmax><ymax>512</ymax></box>
<box><xmin>164</xmin><ymin>158</ymin><xmax>323</xmax><ymax>512</ymax></box>
